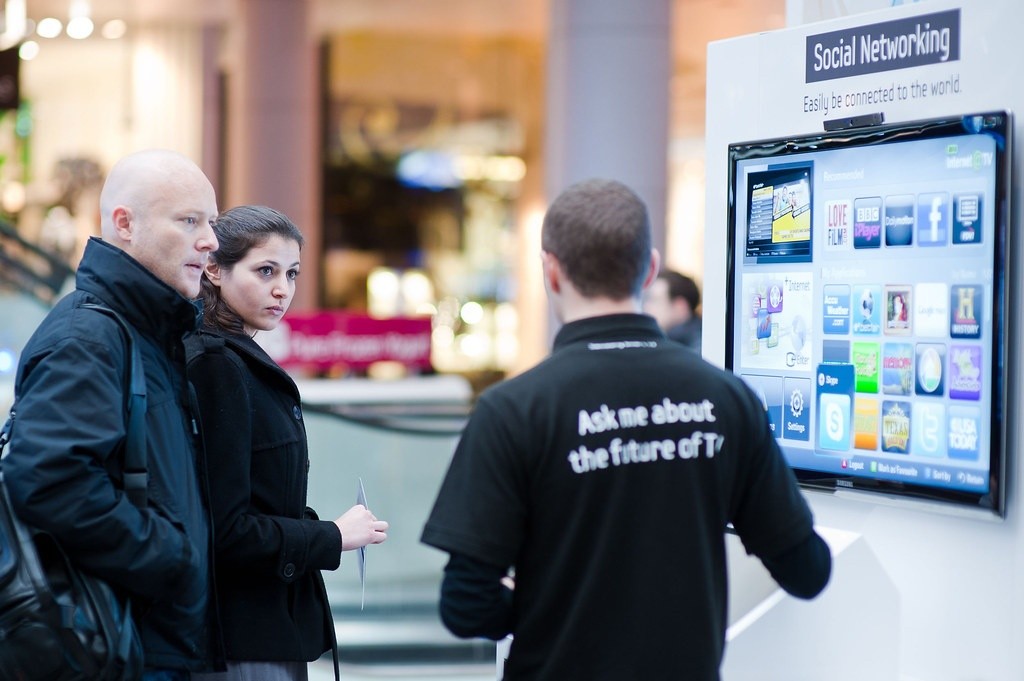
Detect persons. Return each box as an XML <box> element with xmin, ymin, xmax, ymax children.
<box><xmin>185</xmin><ymin>206</ymin><xmax>390</xmax><ymax>681</ymax></box>
<box><xmin>0</xmin><ymin>151</ymin><xmax>222</xmax><ymax>677</ymax></box>
<box><xmin>892</xmin><ymin>294</ymin><xmax>908</xmax><ymax>321</ymax></box>
<box><xmin>422</xmin><ymin>182</ymin><xmax>831</xmax><ymax>681</ymax></box>
<box><xmin>641</xmin><ymin>267</ymin><xmax>703</xmax><ymax>359</ymax></box>
<box><xmin>774</xmin><ymin>186</ymin><xmax>796</xmax><ymax>213</ymax></box>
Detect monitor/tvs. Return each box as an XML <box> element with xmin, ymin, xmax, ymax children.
<box><xmin>723</xmin><ymin>110</ymin><xmax>1013</xmax><ymax>523</ymax></box>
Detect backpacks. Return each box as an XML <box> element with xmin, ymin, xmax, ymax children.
<box><xmin>0</xmin><ymin>303</ymin><xmax>145</xmax><ymax>681</ymax></box>
